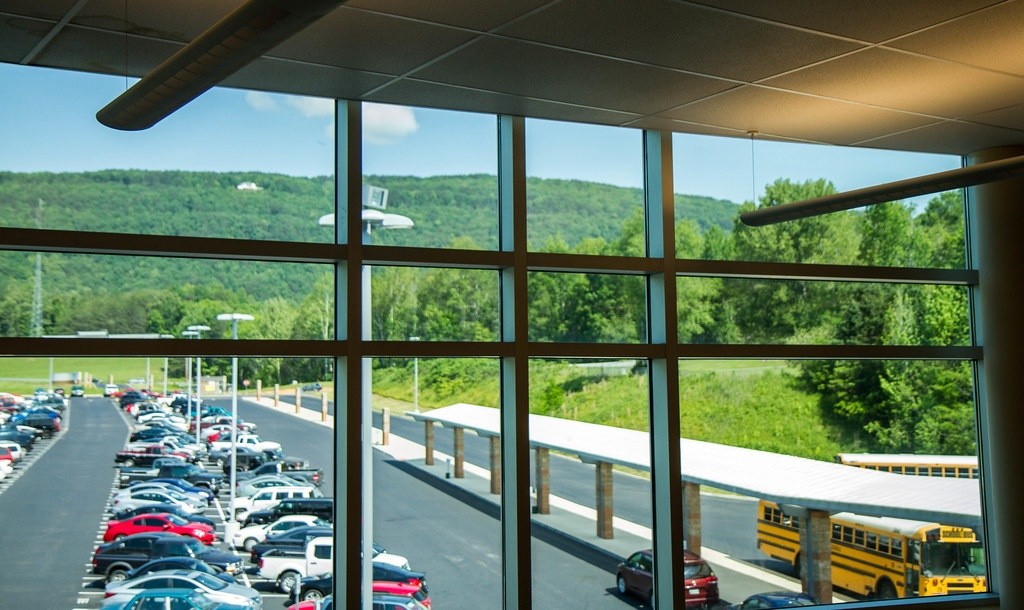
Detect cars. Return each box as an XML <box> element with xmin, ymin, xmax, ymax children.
<box><xmin>721</xmin><ymin>591</ymin><xmax>820</xmax><ymax>610</ymax></box>
<box><xmin>302</xmin><ymin>382</ymin><xmax>321</xmax><ymax>394</ymax></box>
<box><xmin>0</xmin><ymin>379</ymin><xmax>434</xmax><ymax>610</ymax></box>
<box><xmin>616</xmin><ymin>548</ymin><xmax>721</xmax><ymax>610</ymax></box>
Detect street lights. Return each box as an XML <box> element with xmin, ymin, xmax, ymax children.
<box><xmin>187</xmin><ymin>324</ymin><xmax>211</xmax><ymax>445</ymax></box>
<box><xmin>318</xmin><ymin>185</ymin><xmax>415</xmax><ymax>610</ymax></box>
<box><xmin>181</xmin><ymin>330</ymin><xmax>198</xmax><ymax>423</ymax></box>
<box><xmin>217</xmin><ymin>313</ymin><xmax>256</xmax><ymax>522</ymax></box>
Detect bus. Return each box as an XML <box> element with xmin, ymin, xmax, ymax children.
<box><xmin>832</xmin><ymin>453</ymin><xmax>979</xmax><ymax>480</ymax></box>
<box><xmin>757</xmin><ymin>496</ymin><xmax>991</xmax><ymax>602</ymax></box>
